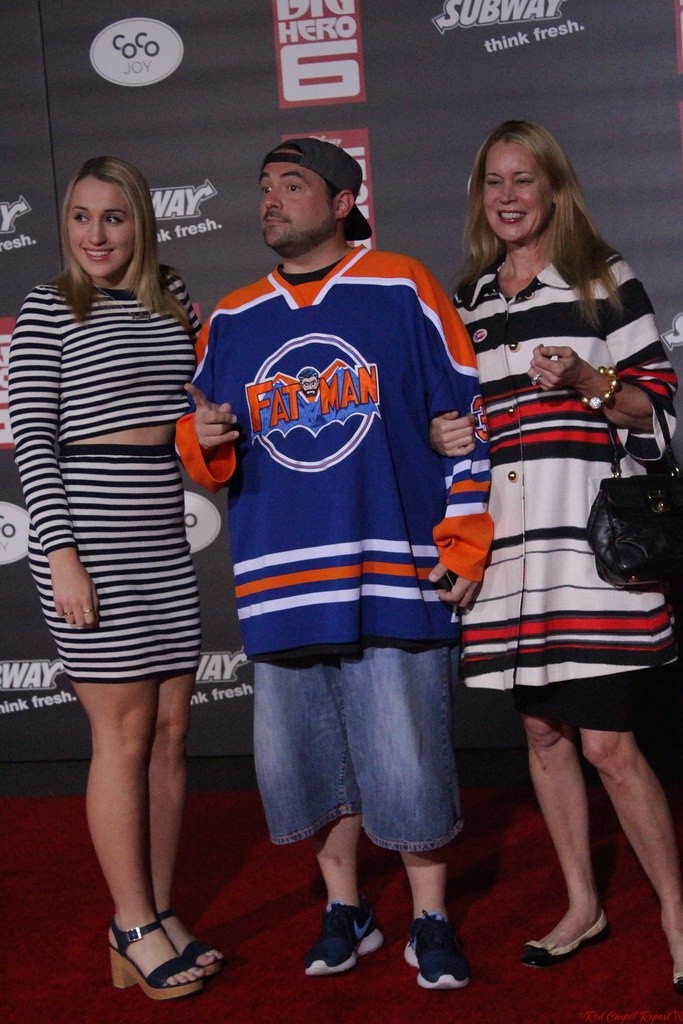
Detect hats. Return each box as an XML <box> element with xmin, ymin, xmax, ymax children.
<box><xmin>263</xmin><ymin>138</ymin><xmax>372</xmax><ymax>241</ymax></box>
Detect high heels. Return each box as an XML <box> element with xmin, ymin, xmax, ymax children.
<box><xmin>107</xmin><ymin>914</ymin><xmax>206</xmax><ymax>999</ymax></box>
<box><xmin>156</xmin><ymin>909</ymin><xmax>223</xmax><ymax>973</ymax></box>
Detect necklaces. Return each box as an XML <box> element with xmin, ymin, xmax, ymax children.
<box><xmin>94</xmin><ymin>283</ymin><xmax>156</xmax><ymax>321</ymax></box>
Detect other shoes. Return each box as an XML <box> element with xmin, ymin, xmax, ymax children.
<box><xmin>672</xmin><ymin>962</ymin><xmax>683</xmax><ymax>994</ymax></box>
<box><xmin>521</xmin><ymin>908</ymin><xmax>608</xmax><ymax>968</ymax></box>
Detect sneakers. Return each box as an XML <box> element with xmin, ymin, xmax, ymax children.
<box><xmin>403</xmin><ymin>910</ymin><xmax>472</xmax><ymax>989</ymax></box>
<box><xmin>304</xmin><ymin>901</ymin><xmax>383</xmax><ymax>975</ymax></box>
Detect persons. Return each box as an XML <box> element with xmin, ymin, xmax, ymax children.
<box><xmin>6</xmin><ymin>156</ymin><xmax>224</xmax><ymax>1002</ymax></box>
<box><xmin>172</xmin><ymin>134</ymin><xmax>496</xmax><ymax>991</ymax></box>
<box><xmin>431</xmin><ymin>118</ymin><xmax>683</xmax><ymax>995</ymax></box>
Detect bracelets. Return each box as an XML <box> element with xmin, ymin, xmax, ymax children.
<box><xmin>582</xmin><ymin>364</ymin><xmax>619</xmax><ymax>411</ymax></box>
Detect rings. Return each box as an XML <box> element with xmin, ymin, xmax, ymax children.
<box><xmin>64</xmin><ymin>612</ymin><xmax>73</xmax><ymax>615</ymax></box>
<box><xmin>530</xmin><ymin>373</ymin><xmax>542</xmax><ymax>386</ymax></box>
<box><xmin>83</xmin><ymin>609</ymin><xmax>92</xmax><ymax>613</ymax></box>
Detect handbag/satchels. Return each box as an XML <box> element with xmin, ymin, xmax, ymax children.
<box><xmin>586</xmin><ymin>381</ymin><xmax>683</xmax><ymax>588</ymax></box>
